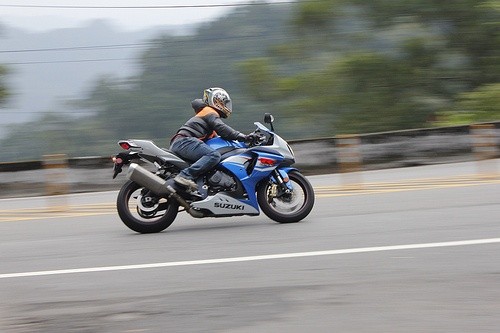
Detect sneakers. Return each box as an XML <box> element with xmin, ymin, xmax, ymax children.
<box><xmin>174</xmin><ymin>175</ymin><xmax>197</xmax><ymax>188</ymax></box>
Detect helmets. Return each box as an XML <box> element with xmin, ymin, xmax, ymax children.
<box><xmin>205</xmin><ymin>85</ymin><xmax>232</xmax><ymax>118</ymax></box>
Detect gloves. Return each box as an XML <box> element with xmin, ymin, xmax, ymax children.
<box><xmin>239</xmin><ymin>132</ymin><xmax>258</xmax><ymax>142</ymax></box>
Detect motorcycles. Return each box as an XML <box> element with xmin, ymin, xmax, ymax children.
<box><xmin>111</xmin><ymin>113</ymin><xmax>316</xmax><ymax>233</ymax></box>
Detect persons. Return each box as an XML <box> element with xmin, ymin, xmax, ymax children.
<box><xmin>170</xmin><ymin>86</ymin><xmax>260</xmax><ymax>188</ymax></box>
